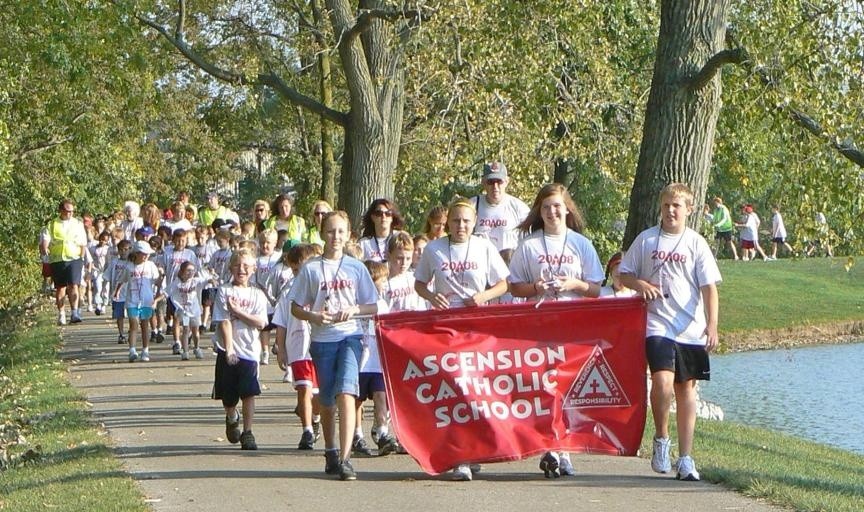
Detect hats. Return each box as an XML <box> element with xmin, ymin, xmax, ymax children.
<box><xmin>134</xmin><ymin>240</ymin><xmax>155</xmax><ymax>254</ymax></box>
<box><xmin>484</xmin><ymin>162</ymin><xmax>507</xmax><ymax>180</ymax></box>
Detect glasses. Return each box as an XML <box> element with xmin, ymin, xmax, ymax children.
<box><xmin>314</xmin><ymin>212</ymin><xmax>326</xmax><ymax>216</ymax></box>
<box><xmin>374</xmin><ymin>210</ymin><xmax>393</xmax><ymax>217</ymax></box>
<box><xmin>486</xmin><ymin>179</ymin><xmax>505</xmax><ymax>185</ymax></box>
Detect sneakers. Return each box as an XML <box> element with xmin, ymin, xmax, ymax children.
<box><xmin>540</xmin><ymin>451</ymin><xmax>574</xmax><ymax>477</ymax></box>
<box><xmin>58</xmin><ymin>301</ymin><xmax>106</xmax><ymax>324</ymax></box>
<box><xmin>284</xmin><ymin>369</ymin><xmax>408</xmax><ymax>480</ymax></box>
<box><xmin>118</xmin><ymin>322</ymin><xmax>206</xmax><ymax>361</ymax></box>
<box><xmin>226</xmin><ymin>408</ymin><xmax>256</xmax><ymax>450</ymax></box>
<box><xmin>261</xmin><ymin>352</ymin><xmax>269</xmax><ymax>364</ymax></box>
<box><xmin>677</xmin><ymin>456</ymin><xmax>700</xmax><ymax>481</ymax></box>
<box><xmin>453</xmin><ymin>463</ymin><xmax>481</xmax><ymax>481</ymax></box>
<box><xmin>652</xmin><ymin>435</ymin><xmax>672</xmax><ymax>473</ymax></box>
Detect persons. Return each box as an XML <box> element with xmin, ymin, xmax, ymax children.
<box><xmin>159</xmin><ymin>192</ymin><xmax>323</xmax><ymax>451</ymax></box>
<box><xmin>769</xmin><ymin>204</ymin><xmax>798</xmax><ymax>260</ymax></box>
<box><xmin>291</xmin><ymin>161</ymin><xmax>634</xmax><ymax>481</ymax></box>
<box><xmin>703</xmin><ymin>197</ymin><xmax>767</xmax><ymax>262</ymax></box>
<box><xmin>616</xmin><ymin>184</ymin><xmax>723</xmax><ymax>479</ymax></box>
<box><xmin>41</xmin><ymin>190</ymin><xmax>198</xmax><ymax>362</ymax></box>
<box><xmin>805</xmin><ymin>210</ymin><xmax>832</xmax><ymax>258</ymax></box>
